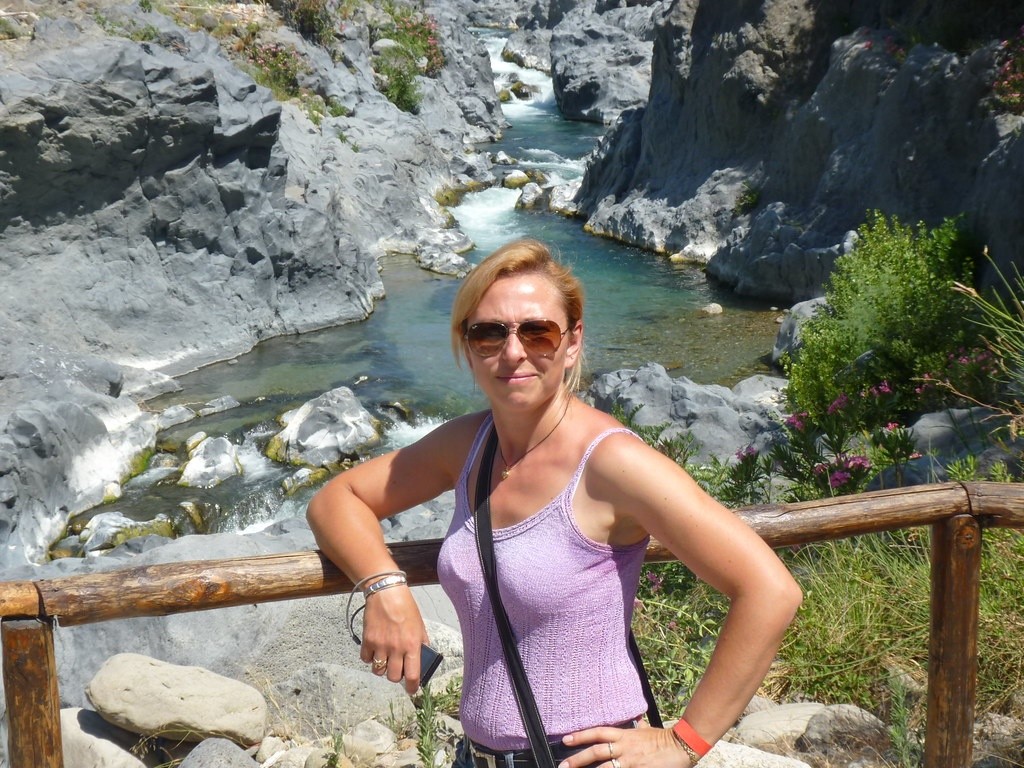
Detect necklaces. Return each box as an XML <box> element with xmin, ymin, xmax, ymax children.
<box><xmin>500</xmin><ymin>397</ymin><xmax>570</xmax><ymax>479</ymax></box>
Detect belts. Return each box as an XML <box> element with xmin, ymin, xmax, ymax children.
<box><xmin>466</xmin><ymin>737</ymin><xmax>567</xmax><ymax>768</ymax></box>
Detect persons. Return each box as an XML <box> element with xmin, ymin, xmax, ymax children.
<box><xmin>305</xmin><ymin>238</ymin><xmax>804</xmax><ymax>768</ymax></box>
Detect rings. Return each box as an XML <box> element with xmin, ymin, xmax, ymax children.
<box><xmin>611</xmin><ymin>759</ymin><xmax>621</xmax><ymax>768</ymax></box>
<box><xmin>372</xmin><ymin>657</ymin><xmax>387</xmax><ymax>670</ymax></box>
<box><xmin>609</xmin><ymin>741</ymin><xmax>613</xmax><ymax>758</ymax></box>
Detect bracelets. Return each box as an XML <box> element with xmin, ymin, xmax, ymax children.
<box><xmin>362</xmin><ymin>576</ymin><xmax>406</xmax><ymax>599</ymax></box>
<box><xmin>673</xmin><ymin>719</ymin><xmax>712</xmax><ymax>766</ymax></box>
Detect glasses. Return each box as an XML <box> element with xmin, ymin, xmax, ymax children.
<box><xmin>463</xmin><ymin>320</ymin><xmax>570</xmax><ymax>357</ymax></box>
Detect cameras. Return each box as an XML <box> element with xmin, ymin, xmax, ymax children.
<box><xmin>419</xmin><ymin>642</ymin><xmax>443</xmax><ymax>687</ymax></box>
